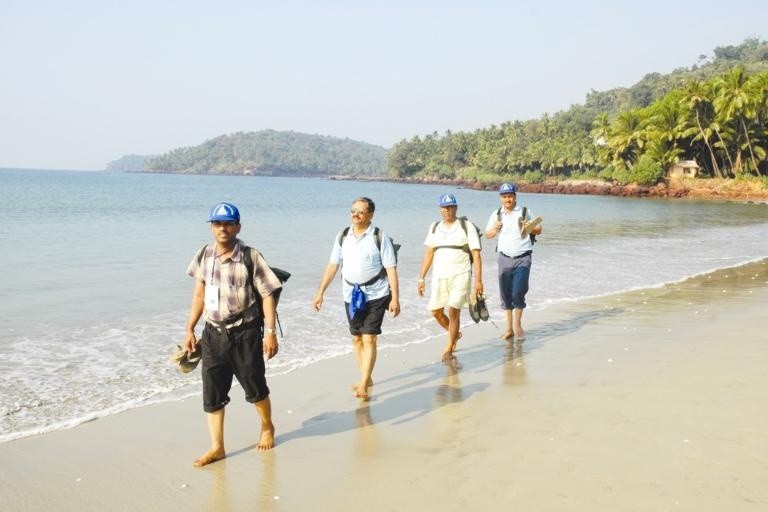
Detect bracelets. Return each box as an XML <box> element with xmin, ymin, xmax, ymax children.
<box><xmin>418</xmin><ymin>278</ymin><xmax>425</xmax><ymax>282</ymax></box>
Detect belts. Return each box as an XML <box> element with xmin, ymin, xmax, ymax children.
<box><xmin>499</xmin><ymin>251</ymin><xmax>533</xmax><ymax>259</ymax></box>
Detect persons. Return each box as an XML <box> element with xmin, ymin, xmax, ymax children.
<box><xmin>187</xmin><ymin>202</ymin><xmax>283</xmax><ymax>467</ymax></box>
<box><xmin>484</xmin><ymin>182</ymin><xmax>541</xmax><ymax>340</ymax></box>
<box><xmin>417</xmin><ymin>193</ymin><xmax>484</xmax><ymax>364</ymax></box>
<box><xmin>313</xmin><ymin>197</ymin><xmax>400</xmax><ymax>399</ymax></box>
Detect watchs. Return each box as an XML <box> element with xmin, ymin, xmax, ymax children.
<box><xmin>263</xmin><ymin>328</ymin><xmax>277</xmax><ymax>334</ymax></box>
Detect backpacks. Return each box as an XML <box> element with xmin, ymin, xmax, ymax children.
<box><xmin>238</xmin><ymin>245</ymin><xmax>290</xmax><ymax>324</ymax></box>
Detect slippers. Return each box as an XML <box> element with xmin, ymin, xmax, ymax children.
<box><xmin>172</xmin><ymin>337</ymin><xmax>203</xmax><ymax>373</ymax></box>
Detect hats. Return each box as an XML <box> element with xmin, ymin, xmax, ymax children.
<box><xmin>206</xmin><ymin>203</ymin><xmax>239</xmax><ymax>226</ymax></box>
<box><xmin>499</xmin><ymin>183</ymin><xmax>515</xmax><ymax>194</ymax></box>
<box><xmin>439</xmin><ymin>193</ymin><xmax>458</xmax><ymax>207</ymax></box>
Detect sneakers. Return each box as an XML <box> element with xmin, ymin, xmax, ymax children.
<box><xmin>468</xmin><ymin>291</ymin><xmax>489</xmax><ymax>323</ymax></box>
